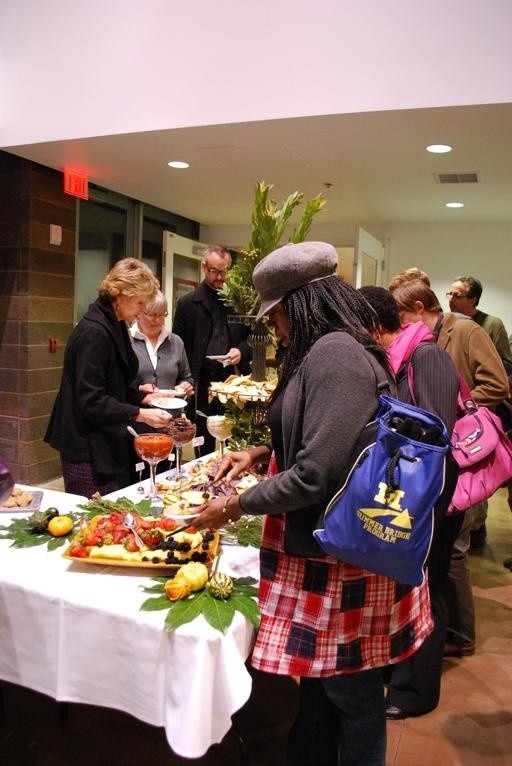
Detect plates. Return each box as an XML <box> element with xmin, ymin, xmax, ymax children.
<box><xmin>0</xmin><ymin>490</ymin><xmax>43</xmax><ymax>513</ymax></box>
<box><xmin>151</xmin><ymin>397</ymin><xmax>188</xmax><ymax>409</ymax></box>
<box><xmin>62</xmin><ymin>529</ymin><xmax>219</xmax><ymax>573</ymax></box>
<box><xmin>205</xmin><ymin>355</ymin><xmax>228</xmax><ymax>360</ymax></box>
<box><xmin>163</xmin><ymin>496</ymin><xmax>218</xmax><ymax>520</ymax></box>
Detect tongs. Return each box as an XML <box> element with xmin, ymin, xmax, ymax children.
<box><xmin>203</xmin><ymin>474</ymin><xmax>228</xmax><ymax>491</ymax></box>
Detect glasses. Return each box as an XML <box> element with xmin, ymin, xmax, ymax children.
<box><xmin>445</xmin><ymin>291</ymin><xmax>470</xmax><ymax>301</ymax></box>
<box><xmin>205</xmin><ymin>263</ymin><xmax>228</xmax><ymax>279</ymax></box>
<box><xmin>143</xmin><ymin>310</ymin><xmax>169</xmax><ymax>318</ymax></box>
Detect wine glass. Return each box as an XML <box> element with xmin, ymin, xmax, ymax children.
<box><xmin>207</xmin><ymin>416</ymin><xmax>231</xmax><ymax>455</ymax></box>
<box><xmin>135</xmin><ymin>433</ymin><xmax>173</xmax><ymax>502</ymax></box>
<box><xmin>165</xmin><ymin>424</ymin><xmax>196</xmax><ymax>481</ymax></box>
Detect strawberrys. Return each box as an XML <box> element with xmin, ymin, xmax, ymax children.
<box><xmin>118</xmin><ymin>511</ymin><xmax>145</xmax><ymax>534</ymax></box>
<box><xmin>70</xmin><ymin>543</ymin><xmax>90</xmax><ymax>558</ymax></box>
<box><xmin>145</xmin><ymin>517</ymin><xmax>177</xmax><ymax>531</ymax></box>
<box><xmin>84</xmin><ymin>516</ymin><xmax>111</xmax><ymax>546</ymax></box>
<box><xmin>119</xmin><ymin>533</ymin><xmax>139</xmax><ymax>552</ymax></box>
<box><xmin>111</xmin><ymin>512</ymin><xmax>119</xmax><ymax>545</ymax></box>
<box><xmin>145</xmin><ymin>529</ymin><xmax>163</xmax><ymax>546</ymax></box>
<box><xmin>184</xmin><ymin>526</ymin><xmax>197</xmax><ymax>534</ymax></box>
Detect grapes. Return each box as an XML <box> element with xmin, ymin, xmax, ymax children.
<box><xmin>142</xmin><ymin>556</ymin><xmax>148</xmax><ymax>562</ymax></box>
<box><xmin>168</xmin><ymin>536</ymin><xmax>191</xmax><ymax>552</ymax></box>
<box><xmin>151</xmin><ymin>556</ymin><xmax>160</xmax><ymax>562</ymax></box>
<box><xmin>164</xmin><ymin>552</ymin><xmax>191</xmax><ymax>565</ymax></box>
<box><xmin>151</xmin><ymin>545</ymin><xmax>158</xmax><ymax>550</ymax></box>
<box><xmin>191</xmin><ymin>551</ymin><xmax>207</xmax><ymax>563</ymax></box>
<box><xmin>160</xmin><ymin>539</ymin><xmax>167</xmax><ymax>549</ymax></box>
<box><xmin>201</xmin><ymin>532</ymin><xmax>214</xmax><ymax>550</ymax></box>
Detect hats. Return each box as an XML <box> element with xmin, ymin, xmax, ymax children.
<box><xmin>251</xmin><ymin>242</ymin><xmax>338</xmax><ymax>321</ymax></box>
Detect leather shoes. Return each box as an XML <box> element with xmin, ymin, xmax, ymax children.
<box><xmin>442</xmin><ymin>641</ymin><xmax>475</xmax><ymax>656</ymax></box>
<box><xmin>383</xmin><ymin>705</ymin><xmax>409</xmax><ymax>719</ymax></box>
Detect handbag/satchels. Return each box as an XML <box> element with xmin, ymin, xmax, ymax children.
<box><xmin>409</xmin><ymin>348</ymin><xmax>512</xmax><ymax>519</ymax></box>
<box><xmin>311</xmin><ymin>395</ymin><xmax>452</xmax><ymax>587</ymax></box>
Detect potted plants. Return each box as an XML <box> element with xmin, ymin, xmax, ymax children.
<box><xmin>216</xmin><ymin>181</ymin><xmax>328</xmax><ymax>377</ymax></box>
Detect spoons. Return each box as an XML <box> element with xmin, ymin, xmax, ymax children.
<box><xmin>122</xmin><ymin>512</ymin><xmax>144</xmax><ymax>548</ymax></box>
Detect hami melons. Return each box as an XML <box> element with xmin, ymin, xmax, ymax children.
<box><xmin>208</xmin><ymin>571</ymin><xmax>233</xmax><ymax>601</ymax></box>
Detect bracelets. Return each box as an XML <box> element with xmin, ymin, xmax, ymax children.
<box><xmin>222</xmin><ymin>495</ymin><xmax>240</xmax><ymax>526</ymax></box>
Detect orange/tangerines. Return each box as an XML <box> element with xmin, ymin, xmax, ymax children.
<box><xmin>47</xmin><ymin>515</ymin><xmax>74</xmax><ymax>538</ymax></box>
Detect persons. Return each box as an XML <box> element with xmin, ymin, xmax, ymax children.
<box><xmin>446</xmin><ymin>275</ymin><xmax>508</xmax><ymax>554</ymax></box>
<box><xmin>390</xmin><ymin>284</ymin><xmax>508</xmax><ymax>656</ymax></box>
<box><xmin>128</xmin><ymin>288</ymin><xmax>194</xmax><ymax>474</ymax></box>
<box><xmin>355</xmin><ymin>285</ymin><xmax>465</xmax><ymax>719</ymax></box>
<box><xmin>185</xmin><ymin>240</ymin><xmax>434</xmax><ymax>750</ymax></box>
<box><xmin>172</xmin><ymin>244</ymin><xmax>254</xmax><ymax>458</ymax></box>
<box><xmin>43</xmin><ymin>256</ymin><xmax>174</xmax><ymax>500</ymax></box>
